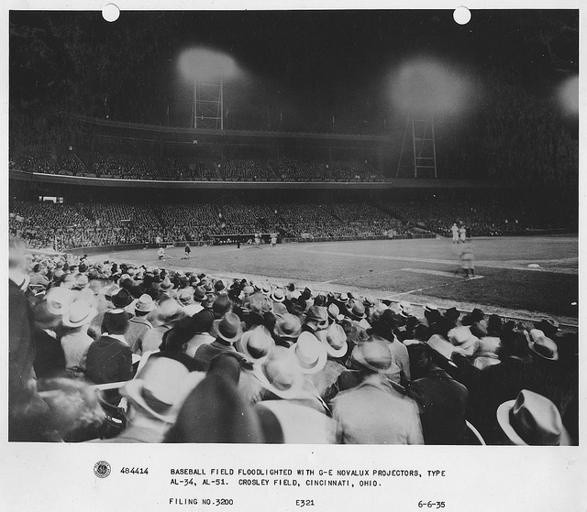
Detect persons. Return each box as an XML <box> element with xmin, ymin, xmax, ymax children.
<box><xmin>8</xmin><ymin>10</ymin><xmax>576</xmax><ymax>243</ymax></box>
<box><xmin>9</xmin><ymin>243</ymin><xmax>247</xmax><ymax>442</ymax></box>
<box><xmin>459</xmin><ymin>247</ymin><xmax>476</xmax><ymax>278</ymax></box>
<box><xmin>247</xmin><ymin>280</ymin><xmax>577</xmax><ymax>445</ymax></box>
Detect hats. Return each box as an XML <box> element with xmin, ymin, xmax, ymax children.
<box><xmin>424</xmin><ymin>303</ymin><xmax>485</xmax><ymax>368</ymax></box>
<box><xmin>46</xmin><ymin>273</ymin><xmax>412</xmax><ymax>446</ymax></box>
<box><xmin>523</xmin><ymin>318</ymin><xmax>562</xmax><ymax>361</ymax></box>
<box><xmin>497</xmin><ymin>389</ymin><xmax>571</xmax><ymax>445</ymax></box>
